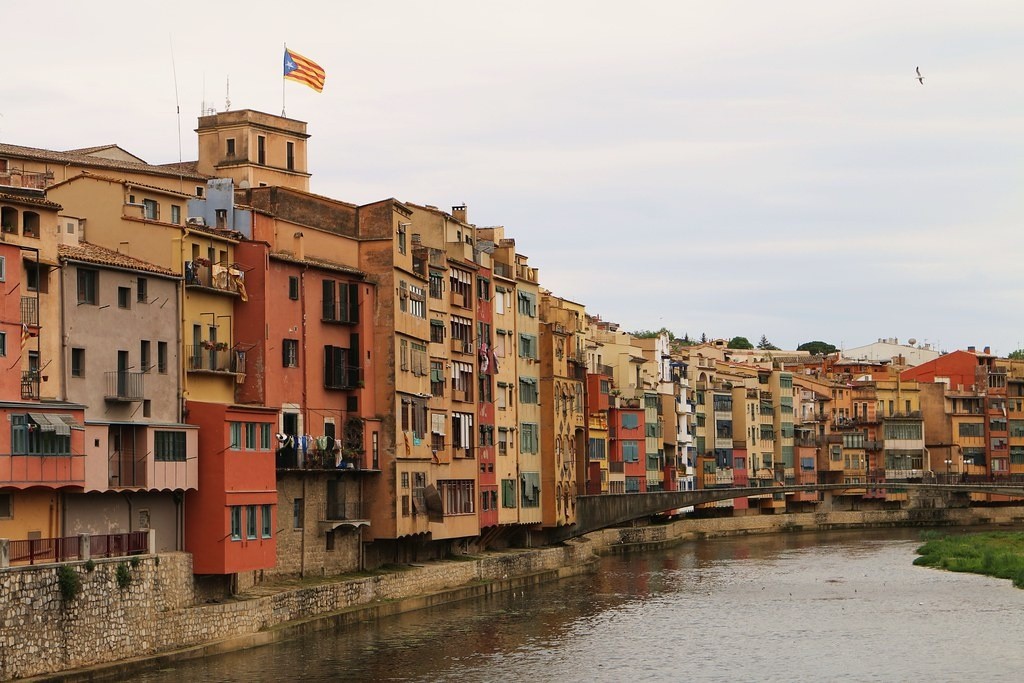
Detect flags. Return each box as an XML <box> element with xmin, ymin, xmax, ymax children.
<box><xmin>284</xmin><ymin>46</ymin><xmax>326</xmax><ymax>93</ymax></box>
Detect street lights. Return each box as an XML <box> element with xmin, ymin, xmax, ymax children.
<box><xmin>963</xmin><ymin>457</ymin><xmax>971</xmax><ymax>474</ymax></box>
<box><xmin>945</xmin><ymin>459</ymin><xmax>952</xmax><ymax>483</ymax></box>
